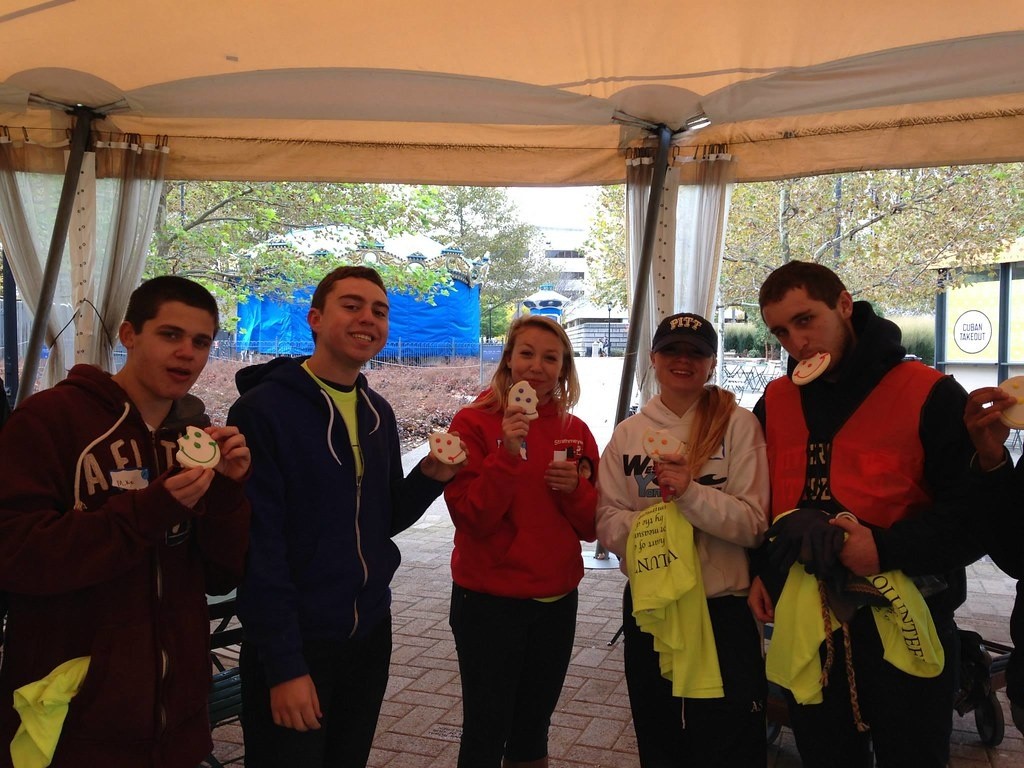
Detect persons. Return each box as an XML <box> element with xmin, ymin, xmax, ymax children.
<box><xmin>747</xmin><ymin>262</ymin><xmax>985</xmax><ymax>768</ymax></box>
<box><xmin>598</xmin><ymin>313</ymin><xmax>768</xmax><ymax>768</ymax></box>
<box><xmin>443</xmin><ymin>317</ymin><xmax>600</xmax><ymax>768</ymax></box>
<box><xmin>226</xmin><ymin>267</ymin><xmax>469</xmax><ymax>768</ymax></box>
<box><xmin>963</xmin><ymin>386</ymin><xmax>1024</xmax><ymax>735</ymax></box>
<box><xmin>0</xmin><ymin>276</ymin><xmax>251</xmax><ymax>768</ymax></box>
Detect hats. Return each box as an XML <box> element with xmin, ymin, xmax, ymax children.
<box><xmin>652</xmin><ymin>313</ymin><xmax>718</xmax><ymax>358</ymax></box>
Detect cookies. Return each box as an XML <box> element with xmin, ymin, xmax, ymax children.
<box><xmin>508</xmin><ymin>380</ymin><xmax>539</xmax><ymax>421</ymax></box>
<box><xmin>643</xmin><ymin>427</ymin><xmax>685</xmax><ymax>464</ymax></box>
<box><xmin>175</xmin><ymin>425</ymin><xmax>220</xmax><ymax>470</ymax></box>
<box><xmin>427</xmin><ymin>432</ymin><xmax>467</xmax><ymax>465</ymax></box>
<box><xmin>993</xmin><ymin>376</ymin><xmax>1024</xmax><ymax>429</ymax></box>
<box><xmin>792</xmin><ymin>352</ymin><xmax>831</xmax><ymax>385</ymax></box>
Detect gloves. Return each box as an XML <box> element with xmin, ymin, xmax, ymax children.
<box><xmin>762</xmin><ymin>508</ymin><xmax>844</xmax><ymax>574</ymax></box>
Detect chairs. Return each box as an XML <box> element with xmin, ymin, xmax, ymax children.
<box><xmin>207</xmin><ymin>597</ymin><xmax>246</xmax><ymax>767</ymax></box>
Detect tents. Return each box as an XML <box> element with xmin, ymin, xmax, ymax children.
<box><xmin>0</xmin><ymin>0</ymin><xmax>1024</xmax><ymax>561</ymax></box>
